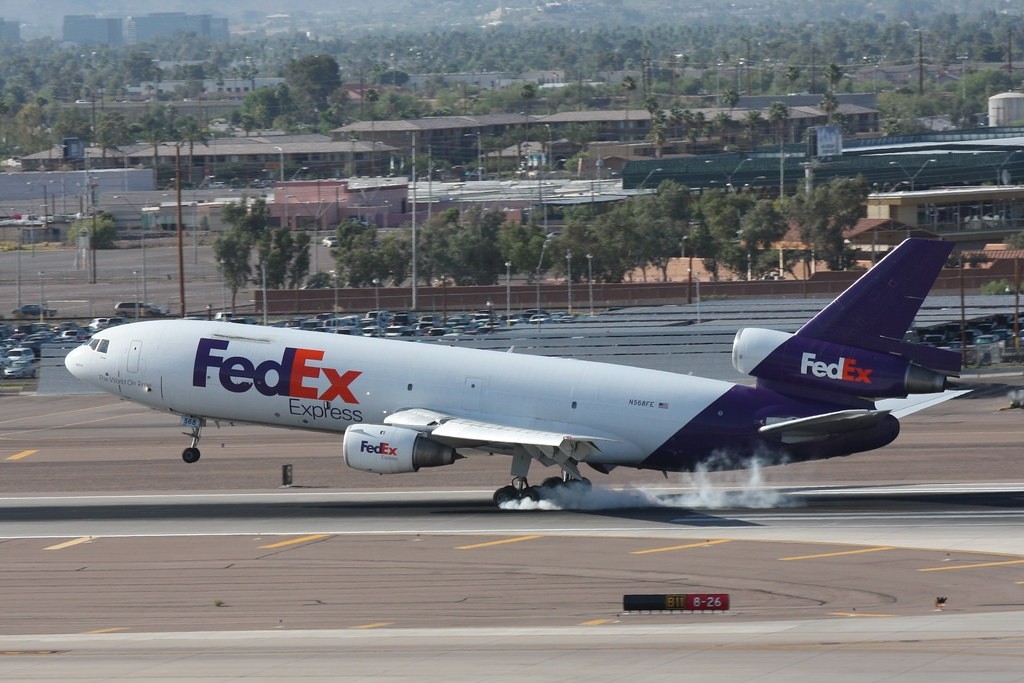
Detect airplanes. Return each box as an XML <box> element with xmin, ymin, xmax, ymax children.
<box><xmin>64</xmin><ymin>238</ymin><xmax>962</xmax><ymax>513</ymax></box>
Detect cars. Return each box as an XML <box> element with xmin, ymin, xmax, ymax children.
<box><xmin>905</xmin><ymin>314</ymin><xmax>1024</xmax><ymax>366</ymax></box>
<box><xmin>185</xmin><ymin>291</ymin><xmax>585</xmax><ymax>364</ymax></box>
<box><xmin>11</xmin><ymin>303</ymin><xmax>57</xmax><ymax>317</ymax></box>
<box><xmin>0</xmin><ymin>317</ymin><xmax>130</xmax><ymax>378</ymax></box>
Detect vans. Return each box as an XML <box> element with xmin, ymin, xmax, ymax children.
<box><xmin>115</xmin><ymin>300</ymin><xmax>161</xmax><ymax>319</ymax></box>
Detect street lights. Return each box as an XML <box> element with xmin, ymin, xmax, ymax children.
<box><xmin>274</xmin><ymin>146</ymin><xmax>284</xmax><ymax>181</ymax></box>
<box><xmin>464</xmin><ymin>131</ymin><xmax>482</xmax><ymax>180</ymax></box>
<box><xmin>220</xmin><ymin>259</ymin><xmax>227</xmax><ymax>321</ymax></box>
<box><xmin>9</xmin><ymin>171</ymin><xmax>54</xmax><ymax>254</ymax></box>
<box><xmin>970</xmin><ymin>149</ymin><xmax>1024</xmax><ymax>187</ymax></box>
<box><xmin>890</xmin><ymin>159</ymin><xmax>937</xmax><ymax>191</ymax></box>
<box><xmin>705</xmin><ymin>159</ymin><xmax>754</xmax><ymax>195</ymax></box>
<box><xmin>133</xmin><ymin>270</ymin><xmax>140</xmax><ymax>320</ymax></box>
<box><xmin>113</xmin><ymin>190</ymin><xmax>170</xmax><ymax>301</ymax></box>
<box><xmin>610</xmin><ymin>168</ymin><xmax>664</xmax><ymax>193</ymax></box>
